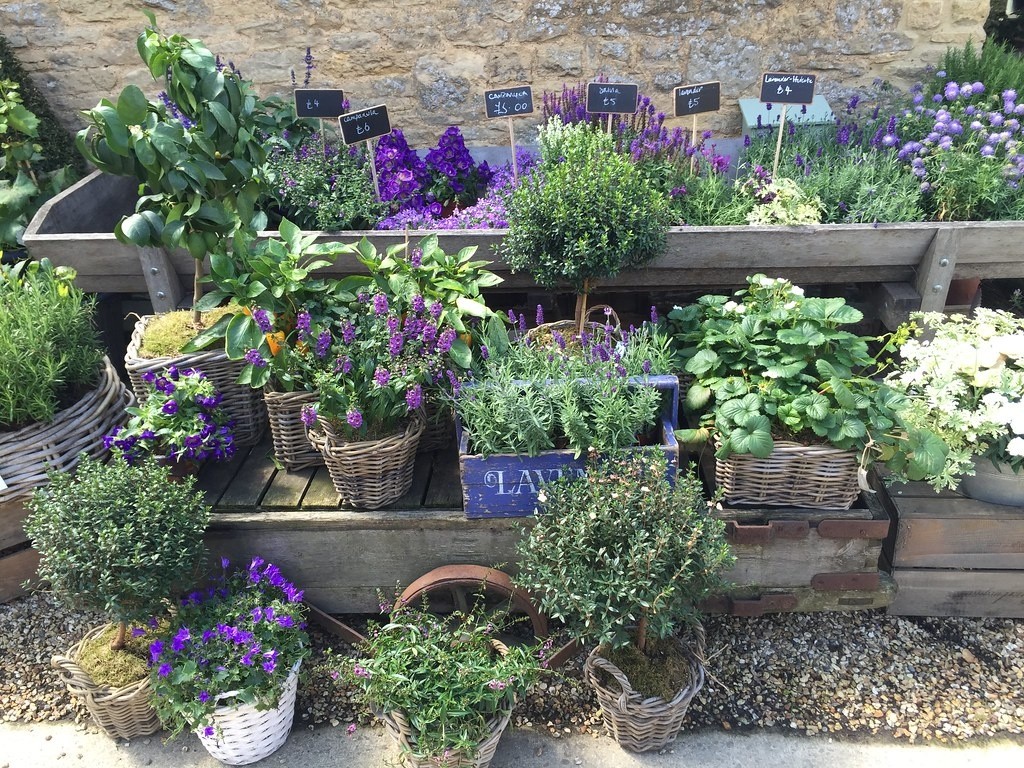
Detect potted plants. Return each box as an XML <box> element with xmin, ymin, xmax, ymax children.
<box><xmin>23</xmin><ymin>451</ymin><xmax>213</xmax><ymax>746</ymax></box>
<box><xmin>503</xmin><ymin>131</ymin><xmax>666</xmax><ymax>349</ymax></box>
<box><xmin>509</xmin><ymin>448</ymin><xmax>724</xmax><ymax>756</ymax></box>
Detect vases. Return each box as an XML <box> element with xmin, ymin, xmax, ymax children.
<box><xmin>23</xmin><ymin>129</ymin><xmax>1024</xmax><ymax>334</ymax></box>
<box><xmin>0</xmin><ymin>356</ymin><xmax>1023</xmax><ymax>768</ymax></box>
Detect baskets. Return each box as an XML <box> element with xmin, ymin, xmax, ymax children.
<box><xmin>124</xmin><ymin>307</ymin><xmax>452</xmax><ymax>510</ymax></box>
<box><xmin>713</xmin><ymin>431</ymin><xmax>867</xmax><ymax>511</ymax></box>
<box><xmin>382</xmin><ymin>639</ymin><xmax>516</xmax><ymax>768</ymax></box>
<box><xmin>522</xmin><ymin>304</ymin><xmax>621</xmax><ymax>357</ymax></box>
<box><xmin>586</xmin><ymin>613</ymin><xmax>705</xmax><ymax>753</ymax></box>
<box><xmin>0</xmin><ymin>349</ymin><xmax>136</xmax><ymax>503</ymax></box>
<box><xmin>168</xmin><ymin>638</ymin><xmax>304</xmax><ymax>765</ymax></box>
<box><xmin>50</xmin><ymin>597</ymin><xmax>179</xmax><ymax>740</ymax></box>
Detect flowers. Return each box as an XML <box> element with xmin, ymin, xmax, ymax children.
<box><xmin>132</xmin><ymin>549</ymin><xmax>330</xmax><ymax>741</ymax></box>
<box><xmin>24</xmin><ymin>48</ymin><xmax>1024</xmax><ymax>505</ymax></box>
